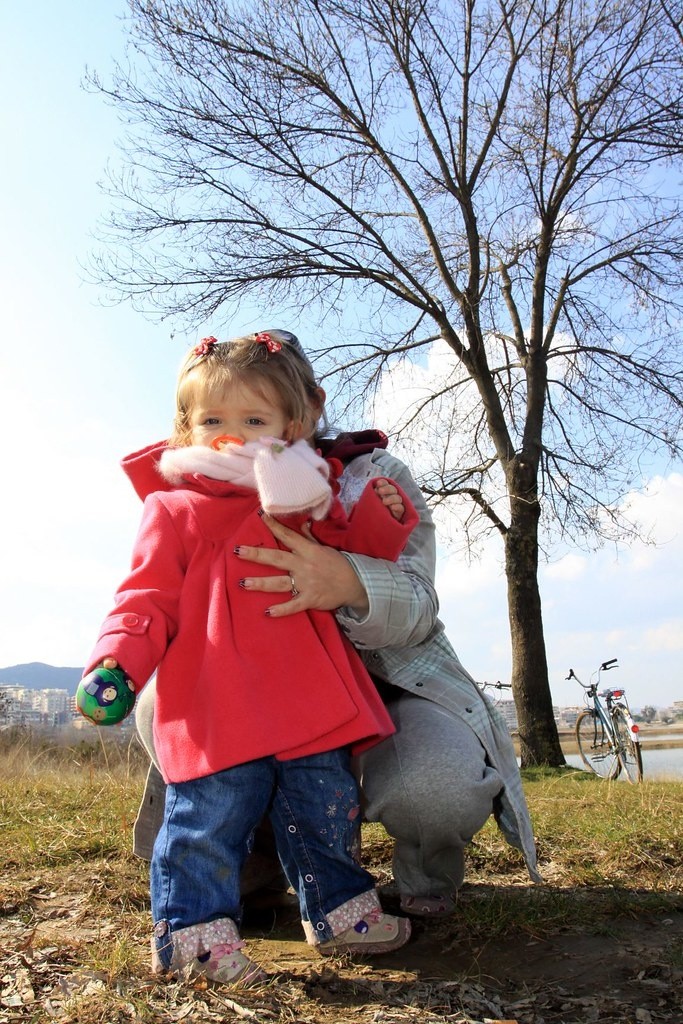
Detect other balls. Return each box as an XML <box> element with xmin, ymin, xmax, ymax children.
<box><xmin>77</xmin><ymin>670</ymin><xmax>139</xmax><ymax>724</ymax></box>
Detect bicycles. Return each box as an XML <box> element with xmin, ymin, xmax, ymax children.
<box><xmin>476</xmin><ymin>681</ymin><xmax>512</xmax><ymax>692</ymax></box>
<box><xmin>565</xmin><ymin>658</ymin><xmax>643</xmax><ymax>785</ymax></box>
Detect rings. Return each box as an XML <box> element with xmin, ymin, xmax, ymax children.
<box><xmin>292</xmin><ymin>577</ymin><xmax>298</xmax><ymax>596</ymax></box>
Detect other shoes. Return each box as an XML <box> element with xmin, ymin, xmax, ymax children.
<box><xmin>318</xmin><ymin>911</ymin><xmax>411</xmax><ymax>954</ymax></box>
<box><xmin>401</xmin><ymin>884</ymin><xmax>461</xmax><ymax>914</ymax></box>
<box><xmin>166</xmin><ymin>939</ymin><xmax>266</xmax><ymax>988</ymax></box>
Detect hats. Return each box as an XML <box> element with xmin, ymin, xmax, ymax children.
<box><xmin>258</xmin><ymin>329</ymin><xmax>312</xmax><ymax>369</ymax></box>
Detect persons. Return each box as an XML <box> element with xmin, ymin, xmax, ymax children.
<box><xmin>77</xmin><ymin>333</ymin><xmax>412</xmax><ymax>991</ymax></box>
<box><xmin>132</xmin><ymin>328</ymin><xmax>542</xmax><ymax>921</ymax></box>
